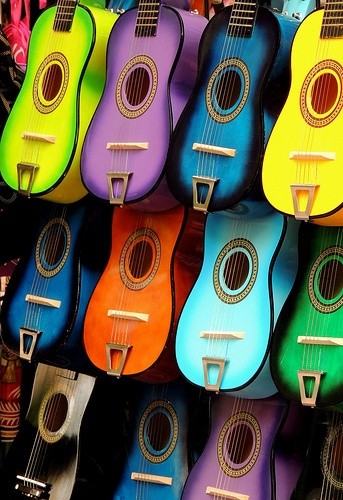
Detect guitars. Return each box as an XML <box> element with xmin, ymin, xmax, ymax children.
<box><xmin>268</xmin><ymin>221</ymin><xmax>343</xmax><ymax>404</ymax></box>
<box><xmin>1</xmin><ymin>362</ymin><xmax>89</xmax><ymax>500</ymax></box>
<box><xmin>166</xmin><ymin>0</ymin><xmax>302</xmax><ymax>219</ymax></box>
<box><xmin>181</xmin><ymin>390</ymin><xmax>303</xmax><ymax>500</ymax></box>
<box><xmin>91</xmin><ymin>366</ymin><xmax>207</xmax><ymax>500</ymax></box>
<box><xmin>260</xmin><ymin>0</ymin><xmax>343</xmax><ymax>227</ymax></box>
<box><xmin>289</xmin><ymin>406</ymin><xmax>343</xmax><ymax>499</ymax></box>
<box><xmin>0</xmin><ymin>201</ymin><xmax>113</xmax><ymax>362</ymax></box>
<box><xmin>79</xmin><ymin>1</ymin><xmax>212</xmax><ymax>212</ymax></box>
<box><xmin>174</xmin><ymin>200</ymin><xmax>306</xmax><ymax>400</ymax></box>
<box><xmin>0</xmin><ymin>0</ymin><xmax>120</xmax><ymax>203</ymax></box>
<box><xmin>83</xmin><ymin>182</ymin><xmax>209</xmax><ymax>382</ymax></box>
